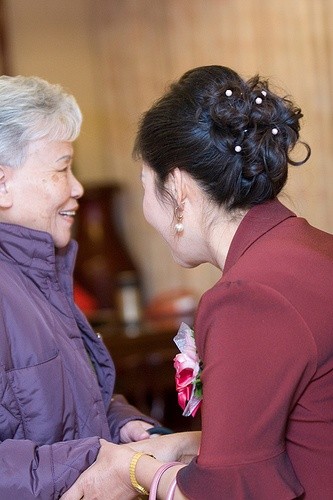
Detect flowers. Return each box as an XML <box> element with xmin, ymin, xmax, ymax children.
<box><xmin>173</xmin><ymin>322</ymin><xmax>201</xmax><ymax>418</ymax></box>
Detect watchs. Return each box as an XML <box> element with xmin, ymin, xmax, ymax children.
<box><xmin>129</xmin><ymin>451</ymin><xmax>158</xmax><ymax>496</ymax></box>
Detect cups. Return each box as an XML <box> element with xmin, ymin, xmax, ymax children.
<box><xmin>113</xmin><ymin>270</ymin><xmax>142</xmax><ymax>325</ymax></box>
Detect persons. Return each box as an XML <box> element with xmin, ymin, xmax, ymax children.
<box><xmin>59</xmin><ymin>65</ymin><xmax>333</xmax><ymax>500</ymax></box>
<box><xmin>1</xmin><ymin>74</ymin><xmax>165</xmax><ymax>500</ymax></box>
